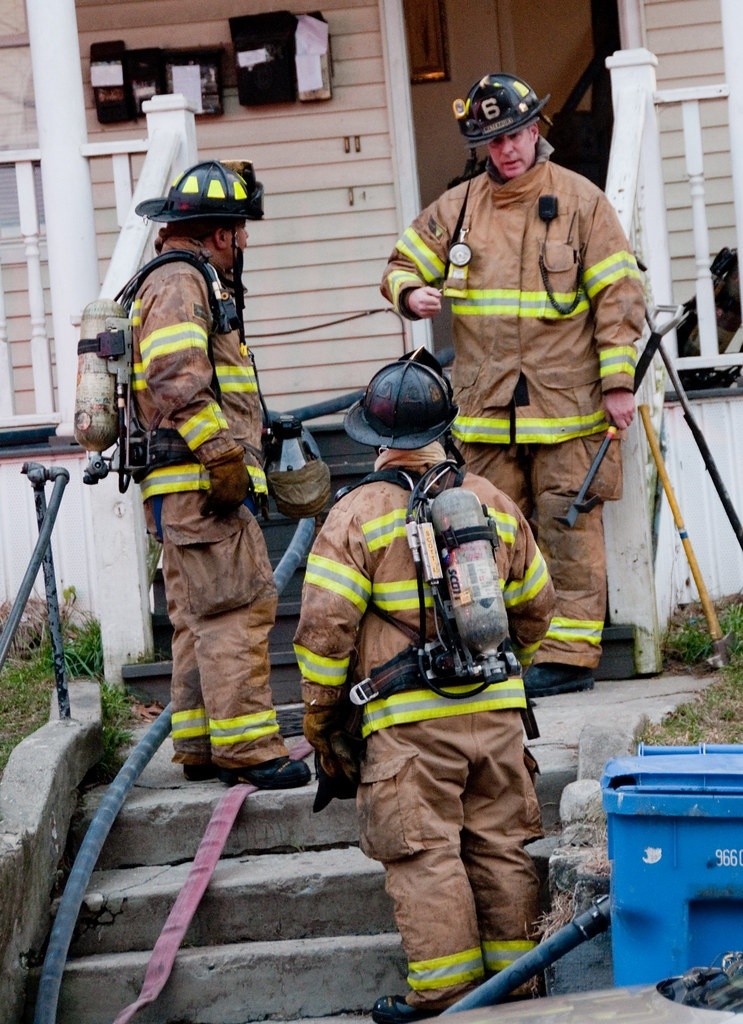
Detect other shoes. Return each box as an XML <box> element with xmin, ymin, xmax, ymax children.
<box><xmin>521</xmin><ymin>663</ymin><xmax>594</xmax><ymax>697</ymax></box>
<box><xmin>183</xmin><ymin>761</ymin><xmax>220</xmax><ymax>781</ymax></box>
<box><xmin>216</xmin><ymin>756</ymin><xmax>311</xmax><ymax>788</ymax></box>
<box><xmin>372</xmin><ymin>995</ymin><xmax>445</xmax><ymax>1024</ymax></box>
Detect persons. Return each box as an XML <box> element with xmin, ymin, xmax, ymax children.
<box><xmin>291</xmin><ymin>349</ymin><xmax>557</xmax><ymax>1024</ymax></box>
<box><xmin>380</xmin><ymin>73</ymin><xmax>644</xmax><ymax>701</ymax></box>
<box><xmin>117</xmin><ymin>161</ymin><xmax>310</xmax><ymax>790</ymax></box>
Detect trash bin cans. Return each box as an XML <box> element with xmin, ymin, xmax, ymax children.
<box><xmin>604</xmin><ymin>742</ymin><xmax>743</xmax><ymax>990</ymax></box>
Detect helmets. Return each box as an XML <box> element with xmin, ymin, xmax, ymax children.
<box><xmin>459</xmin><ymin>72</ymin><xmax>548</xmax><ymax>150</ymax></box>
<box><xmin>135</xmin><ymin>160</ymin><xmax>263</xmax><ymax>222</ymax></box>
<box><xmin>344</xmin><ymin>360</ymin><xmax>460</xmax><ymax>450</ymax></box>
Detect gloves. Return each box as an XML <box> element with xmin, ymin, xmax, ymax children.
<box><xmin>199</xmin><ymin>445</ymin><xmax>250</xmax><ymax>516</ymax></box>
<box><xmin>303</xmin><ymin>710</ymin><xmax>340</xmax><ymax>754</ymax></box>
